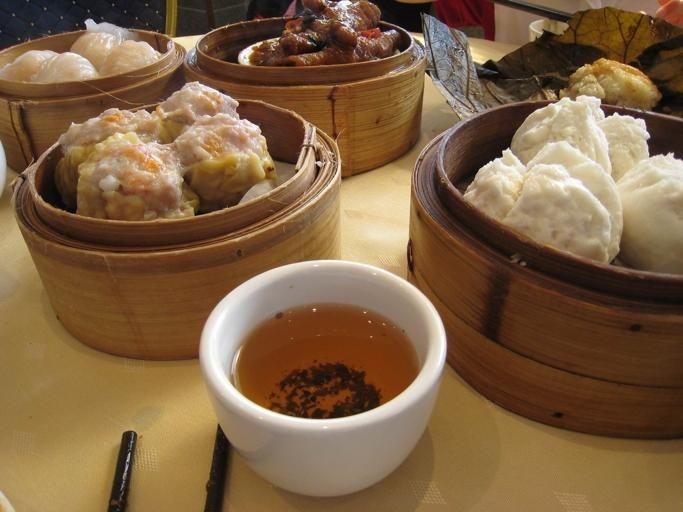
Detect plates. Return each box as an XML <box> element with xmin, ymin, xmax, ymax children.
<box><xmin>237</xmin><ymin>38</ymin><xmax>402</xmax><ymax>67</ymax></box>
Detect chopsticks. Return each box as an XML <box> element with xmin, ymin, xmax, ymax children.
<box><xmin>108</xmin><ymin>423</ymin><xmax>243</xmax><ymax>511</ymax></box>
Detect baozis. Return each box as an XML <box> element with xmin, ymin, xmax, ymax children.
<box><xmin>3</xmin><ymin>19</ymin><xmax>162</xmax><ymax>83</ymax></box>
<box><xmin>463</xmin><ymin>95</ymin><xmax>682</xmax><ymax>273</ymax></box>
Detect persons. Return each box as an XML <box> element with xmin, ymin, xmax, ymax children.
<box><xmin>652</xmin><ymin>0</ymin><xmax>682</xmax><ymax>30</ymax></box>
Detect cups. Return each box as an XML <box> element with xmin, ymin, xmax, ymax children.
<box><xmin>527</xmin><ymin>18</ymin><xmax>571</xmax><ymax>42</ymax></box>
<box><xmin>194</xmin><ymin>259</ymin><xmax>448</xmax><ymax>499</ymax></box>
